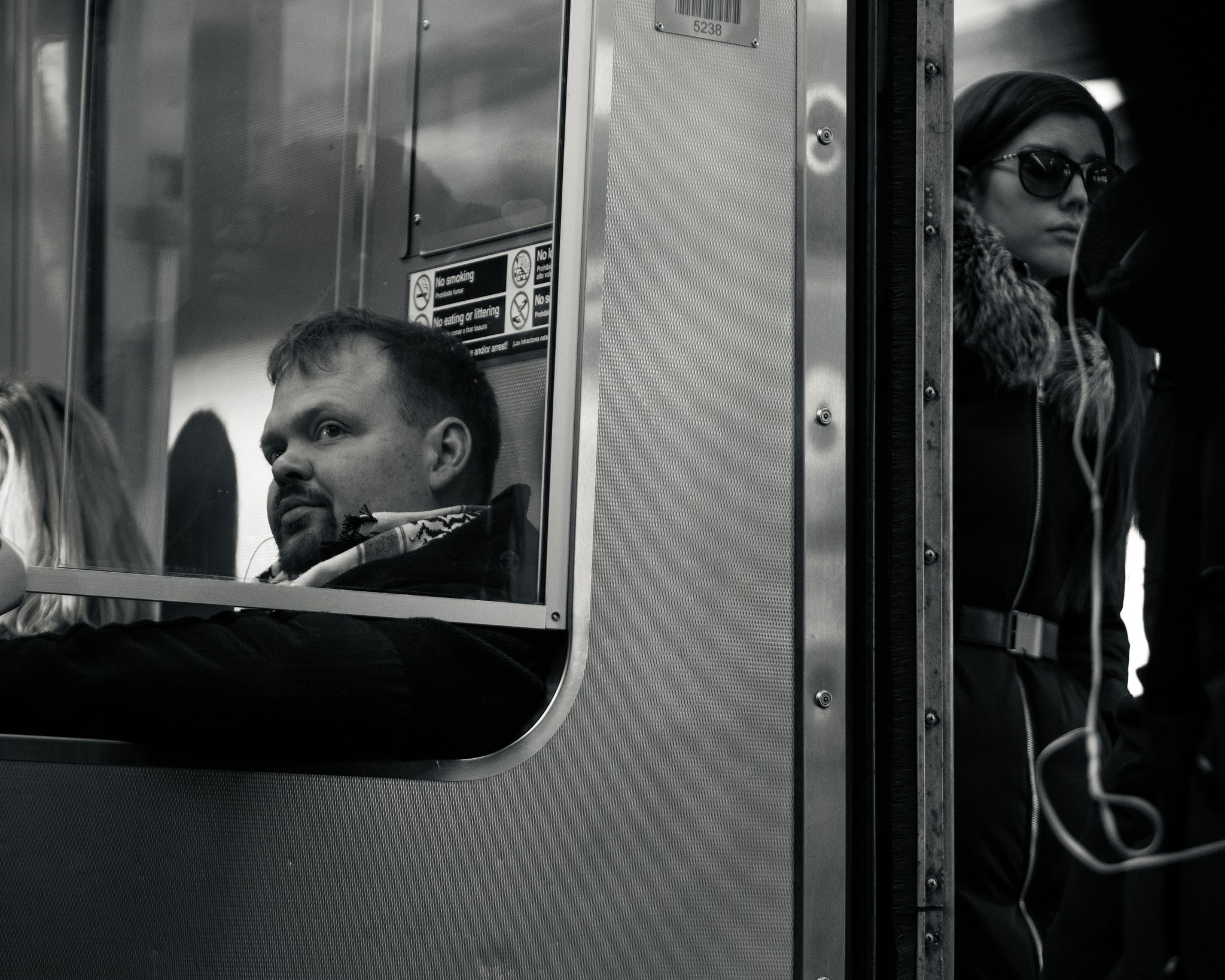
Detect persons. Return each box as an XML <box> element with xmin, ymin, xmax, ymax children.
<box><xmin>0</xmin><ymin>307</ymin><xmax>568</xmax><ymax>765</ymax></box>
<box><xmin>954</xmin><ymin>0</ymin><xmax>1224</xmax><ymax>980</ymax></box>
<box><xmin>0</xmin><ymin>375</ymin><xmax>159</xmax><ymax>640</ymax></box>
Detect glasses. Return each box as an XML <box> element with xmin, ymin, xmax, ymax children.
<box><xmin>988</xmin><ymin>148</ymin><xmax>1125</xmax><ymax>208</ymax></box>
